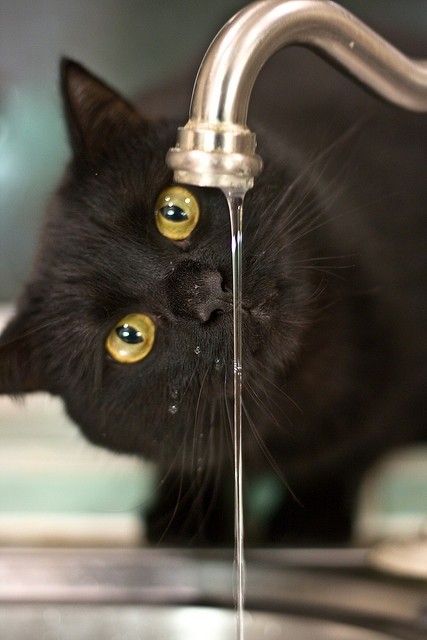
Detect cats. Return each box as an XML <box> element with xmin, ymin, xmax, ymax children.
<box><xmin>0</xmin><ymin>52</ymin><xmax>425</xmax><ymax>549</ymax></box>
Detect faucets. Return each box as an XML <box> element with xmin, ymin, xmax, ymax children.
<box><xmin>164</xmin><ymin>1</ymin><xmax>426</xmax><ymax>188</ymax></box>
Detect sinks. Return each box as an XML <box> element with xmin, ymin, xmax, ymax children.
<box><xmin>0</xmin><ymin>533</ymin><xmax>427</xmax><ymax>640</ymax></box>
